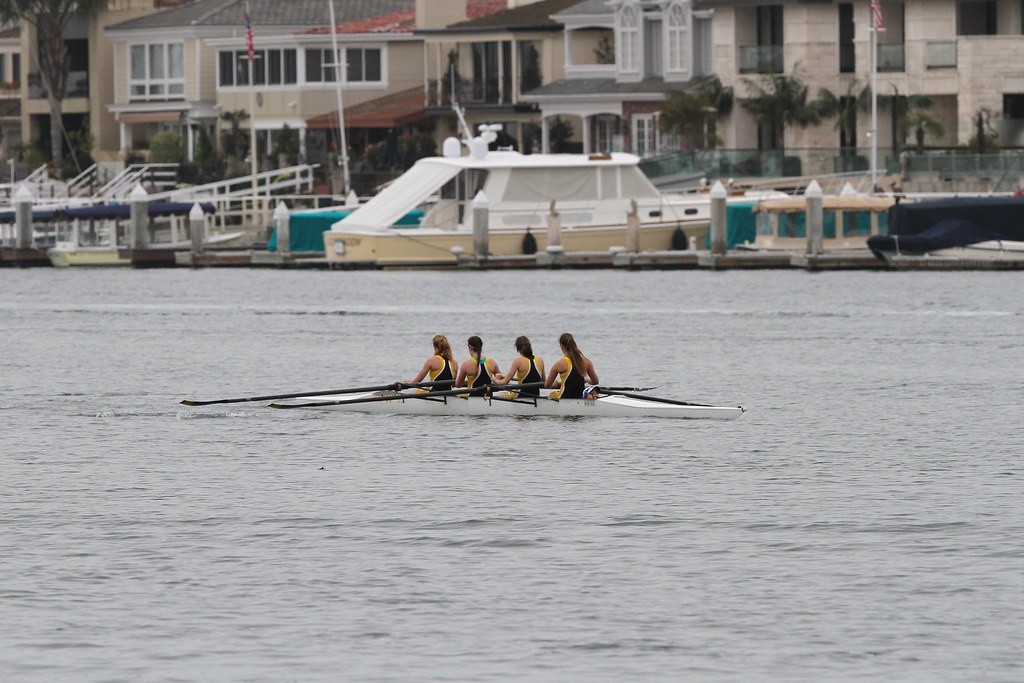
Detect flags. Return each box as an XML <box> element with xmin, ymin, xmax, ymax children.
<box><xmin>871</xmin><ymin>0</ymin><xmax>883</xmax><ymax>27</ymax></box>
<box><xmin>246</xmin><ymin>15</ymin><xmax>255</xmax><ymax>60</ymax></box>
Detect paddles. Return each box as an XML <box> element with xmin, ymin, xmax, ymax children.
<box><xmin>541</xmin><ymin>382</ymin><xmax>713</xmax><ymax>407</ymax></box>
<box><xmin>179</xmin><ymin>377</ymin><xmax>455</xmax><ymax>407</ymax></box>
<box><xmin>266</xmin><ymin>380</ymin><xmax>547</xmax><ymax>409</ymax></box>
<box><xmin>500</xmin><ymin>376</ymin><xmax>658</xmax><ymax>392</ymax></box>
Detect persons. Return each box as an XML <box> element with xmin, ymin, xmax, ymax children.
<box><xmin>403</xmin><ymin>334</ymin><xmax>459</xmax><ymax>394</ymax></box>
<box><xmin>583</xmin><ymin>386</ymin><xmax>601</xmax><ymax>401</ymax></box>
<box><xmin>545</xmin><ymin>333</ymin><xmax>599</xmax><ymax>399</ymax></box>
<box><xmin>454</xmin><ymin>336</ymin><xmax>500</xmax><ymax>397</ymax></box>
<box><xmin>491</xmin><ymin>336</ymin><xmax>546</xmax><ymax>400</ymax></box>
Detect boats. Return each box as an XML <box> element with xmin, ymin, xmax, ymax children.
<box><xmin>320</xmin><ymin>113</ymin><xmax>719</xmax><ymax>266</ymax></box>
<box><xmin>280</xmin><ymin>382</ymin><xmax>748</xmax><ymax>422</ymax></box>
